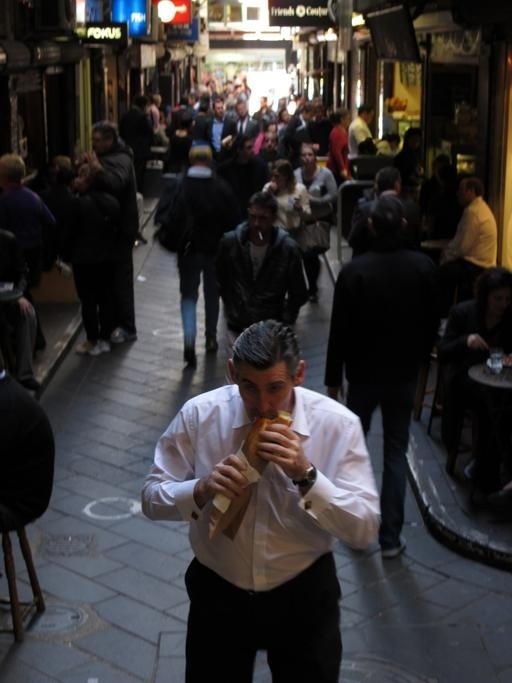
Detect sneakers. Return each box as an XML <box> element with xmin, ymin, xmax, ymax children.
<box><xmin>75</xmin><ymin>326</ymin><xmax>136</xmax><ymax>356</ymax></box>
<box><xmin>382</xmin><ymin>535</ymin><xmax>406</xmax><ymax>556</ymax></box>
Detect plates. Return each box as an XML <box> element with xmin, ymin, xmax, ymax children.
<box><xmin>486</xmin><ymin>358</ymin><xmax>503</xmax><ymax>368</ymax></box>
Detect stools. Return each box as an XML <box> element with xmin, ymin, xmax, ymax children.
<box><xmin>1</xmin><ymin>520</ymin><xmax>45</xmax><ymax>646</ymax></box>
<box><xmin>413</xmin><ymin>315</ymin><xmax>512</xmax><ymax>515</ymax></box>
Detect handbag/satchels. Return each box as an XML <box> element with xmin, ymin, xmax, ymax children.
<box><xmin>293</xmin><ymin>214</ymin><xmax>331</xmax><ymax>254</ymax></box>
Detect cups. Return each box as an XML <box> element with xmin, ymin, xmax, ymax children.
<box><xmin>488</xmin><ymin>348</ymin><xmax>503</xmax><ymax>374</ymax></box>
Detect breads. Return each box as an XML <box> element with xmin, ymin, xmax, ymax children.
<box><xmin>241</xmin><ymin>411</ymin><xmax>293</xmax><ymax>474</ymax></box>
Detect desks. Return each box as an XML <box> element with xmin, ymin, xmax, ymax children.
<box><xmin>0</xmin><ymin>287</ymin><xmax>24</xmax><ymax>302</ymax></box>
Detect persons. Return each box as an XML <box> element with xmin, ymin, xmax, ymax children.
<box><xmin>141</xmin><ymin>318</ymin><xmax>382</xmax><ymax>683</ymax></box>
<box><xmin>323</xmin><ymin>196</ymin><xmax>440</xmax><ymax>558</ymax></box>
<box><xmin>0</xmin><ymin>357</ymin><xmax>56</xmax><ymax>532</ymax></box>
<box><xmin>1</xmin><ymin>121</ymin><xmax>138</xmax><ymax>390</ymax></box>
<box><xmin>117</xmin><ymin>68</ymin><xmax>423</xmax><ymax>370</ymax></box>
<box><xmin>435</xmin><ymin>266</ymin><xmax>512</xmax><ymax>497</ymax></box>
<box><xmin>415</xmin><ymin>129</ymin><xmax>512</xmax><ymax>293</ymax></box>
